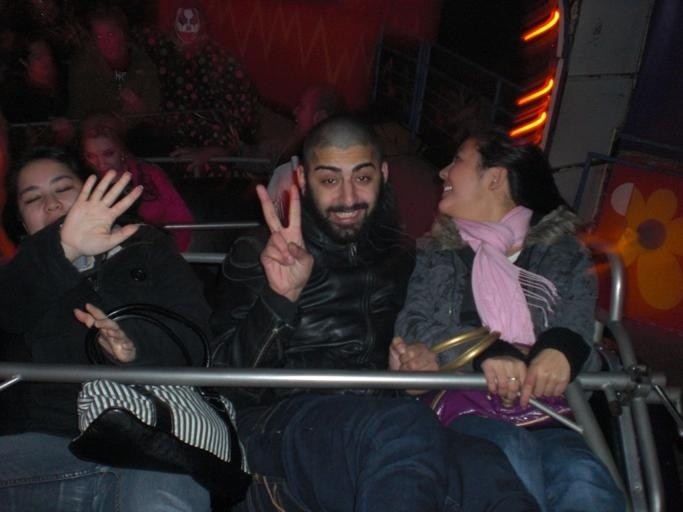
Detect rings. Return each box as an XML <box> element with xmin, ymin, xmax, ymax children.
<box><xmin>507</xmin><ymin>376</ymin><xmax>519</xmax><ymax>381</ymax></box>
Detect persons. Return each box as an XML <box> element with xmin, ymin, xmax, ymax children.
<box><xmin>392</xmin><ymin>125</ymin><xmax>631</xmax><ymax>510</ymax></box>
<box><xmin>0</xmin><ymin>144</ymin><xmax>216</xmax><ymax>512</ymax></box>
<box><xmin>201</xmin><ymin>112</ymin><xmax>542</xmax><ymax>511</ymax></box>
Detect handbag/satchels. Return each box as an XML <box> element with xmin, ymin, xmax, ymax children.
<box><xmin>65</xmin><ymin>303</ymin><xmax>255</xmax><ymax>507</ymax></box>
<box><xmin>406</xmin><ymin>324</ymin><xmax>573</xmax><ymax>433</ymax></box>
